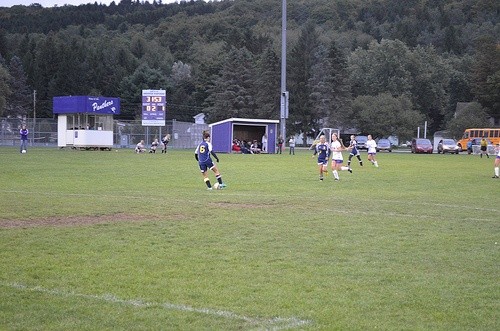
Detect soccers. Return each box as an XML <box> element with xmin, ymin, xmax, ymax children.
<box><xmin>213</xmin><ymin>181</ymin><xmax>222</xmax><ymax>190</ymax></box>
<box><xmin>21</xmin><ymin>150</ymin><xmax>26</xmax><ymax>154</ymax></box>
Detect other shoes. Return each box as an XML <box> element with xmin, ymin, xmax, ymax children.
<box><xmin>219</xmin><ymin>184</ymin><xmax>227</xmax><ymax>190</ymax></box>
<box><xmin>492</xmin><ymin>175</ymin><xmax>499</xmax><ymax>178</ymax></box>
<box><xmin>348</xmin><ymin>168</ymin><xmax>353</xmax><ymax>173</ymax></box>
<box><xmin>334</xmin><ymin>178</ymin><xmax>339</xmax><ymax>181</ymax></box>
<box><xmin>207</xmin><ymin>188</ymin><xmax>213</xmax><ymax>191</ymax></box>
<box><xmin>374</xmin><ymin>160</ymin><xmax>378</xmax><ymax>167</ymax></box>
<box><xmin>320</xmin><ymin>178</ymin><xmax>323</xmax><ymax>181</ymax></box>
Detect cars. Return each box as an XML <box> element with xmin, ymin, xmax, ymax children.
<box><xmin>437</xmin><ymin>139</ymin><xmax>459</xmax><ymax>154</ymax></box>
<box><xmin>411</xmin><ymin>138</ymin><xmax>433</xmax><ymax>153</ymax></box>
<box><xmin>377</xmin><ymin>139</ymin><xmax>392</xmax><ymax>152</ymax></box>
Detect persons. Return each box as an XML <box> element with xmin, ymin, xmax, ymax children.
<box><xmin>277</xmin><ymin>136</ymin><xmax>284</xmax><ymax>154</ymax></box>
<box><xmin>365</xmin><ymin>135</ymin><xmax>379</xmax><ymax>167</ymax></box>
<box><xmin>330</xmin><ymin>133</ymin><xmax>352</xmax><ymax>181</ymax></box>
<box><xmin>195</xmin><ymin>131</ymin><xmax>227</xmax><ymax>190</ymax></box>
<box><xmin>135</xmin><ymin>140</ymin><xmax>146</xmax><ymax>154</ymax></box>
<box><xmin>466</xmin><ymin>139</ymin><xmax>473</xmax><ymax>154</ymax></box>
<box><xmin>312</xmin><ymin>135</ymin><xmax>331</xmax><ymax>181</ymax></box>
<box><xmin>20</xmin><ymin>126</ymin><xmax>29</xmax><ymax>153</ymax></box>
<box><xmin>480</xmin><ymin>139</ymin><xmax>489</xmax><ymax>158</ymax></box>
<box><xmin>348</xmin><ymin>135</ymin><xmax>363</xmax><ymax>167</ymax></box>
<box><xmin>233</xmin><ymin>134</ymin><xmax>267</xmax><ymax>154</ymax></box>
<box><xmin>492</xmin><ymin>146</ymin><xmax>500</xmax><ymax>178</ymax></box>
<box><xmin>148</xmin><ymin>139</ymin><xmax>159</xmax><ymax>153</ymax></box>
<box><xmin>160</xmin><ymin>134</ymin><xmax>171</xmax><ymax>153</ymax></box>
<box><xmin>289</xmin><ymin>136</ymin><xmax>297</xmax><ymax>155</ymax></box>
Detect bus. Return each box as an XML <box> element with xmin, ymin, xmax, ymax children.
<box><xmin>458</xmin><ymin>128</ymin><xmax>500</xmax><ymax>152</ymax></box>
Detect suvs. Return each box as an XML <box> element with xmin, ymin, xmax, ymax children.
<box><xmin>355</xmin><ymin>135</ymin><xmax>369</xmax><ymax>151</ymax></box>
